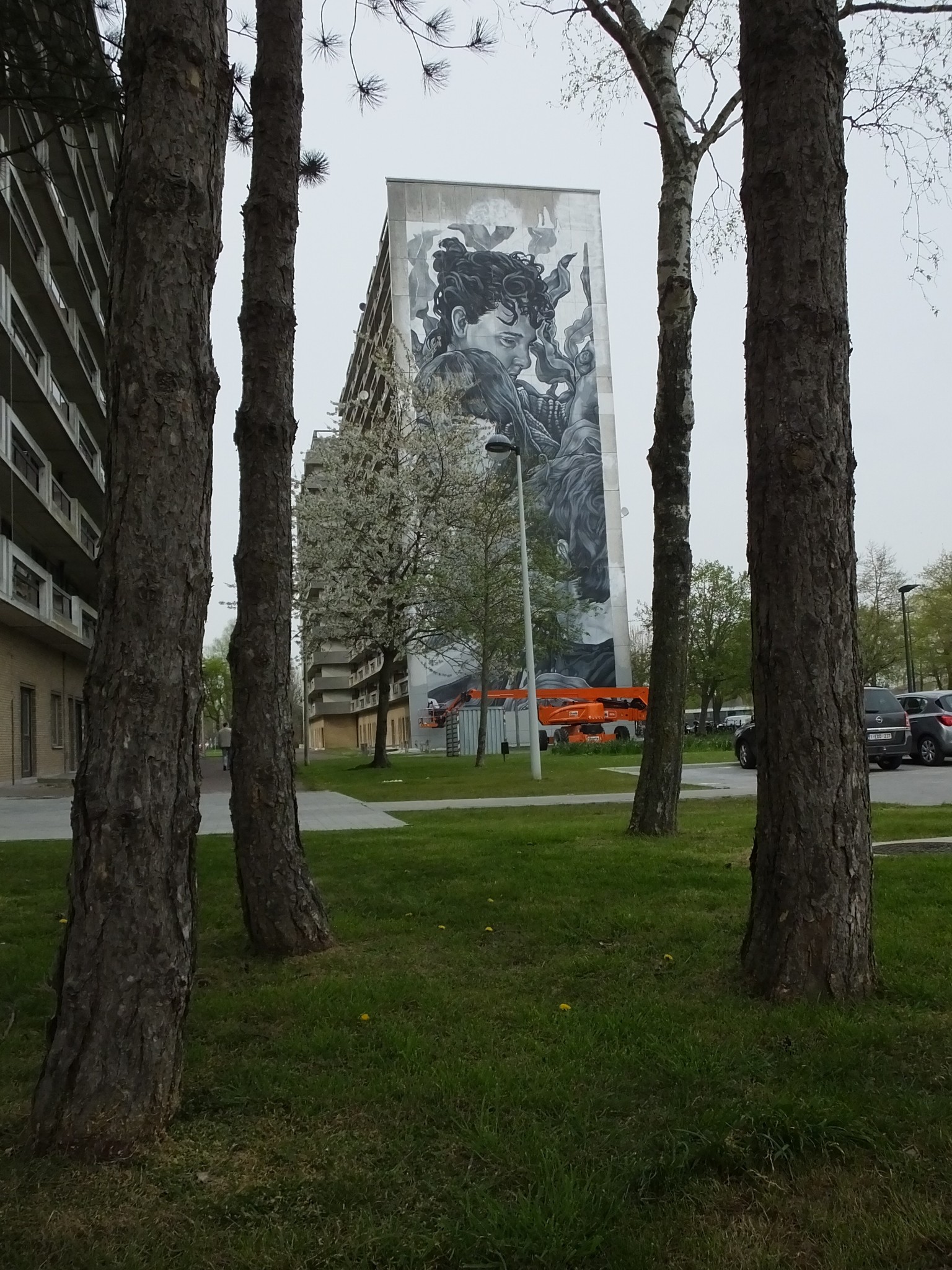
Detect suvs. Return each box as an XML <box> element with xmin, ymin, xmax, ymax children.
<box><xmin>894</xmin><ymin>689</ymin><xmax>952</xmax><ymax>767</ymax></box>
<box><xmin>732</xmin><ymin>684</ymin><xmax>913</xmax><ymax>771</ymax></box>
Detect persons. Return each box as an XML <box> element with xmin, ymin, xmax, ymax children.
<box><xmin>217</xmin><ymin>722</ymin><xmax>232</xmax><ymax>771</ymax></box>
<box><xmin>684</xmin><ymin>722</ymin><xmax>690</xmax><ymax>734</ymax></box>
<box><xmin>429</xmin><ymin>701</ymin><xmax>435</xmax><ymax>716</ymax></box>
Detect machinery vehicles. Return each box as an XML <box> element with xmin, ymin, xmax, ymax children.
<box><xmin>417</xmin><ymin>686</ymin><xmax>649</xmax><ymax>751</ymax></box>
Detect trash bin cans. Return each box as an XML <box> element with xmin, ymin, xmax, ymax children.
<box><xmin>360</xmin><ymin>743</ymin><xmax>368</xmax><ymax>752</ymax></box>
<box><xmin>501</xmin><ymin>742</ymin><xmax>509</xmax><ymax>754</ymax></box>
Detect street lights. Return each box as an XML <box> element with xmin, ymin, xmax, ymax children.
<box><xmin>906</xmin><ymin>610</ymin><xmax>917</xmax><ymax>693</ymax></box>
<box><xmin>897</xmin><ymin>584</ymin><xmax>921</xmax><ymax>695</ymax></box>
<box><xmin>484</xmin><ymin>433</ymin><xmax>542</xmax><ymax>781</ymax></box>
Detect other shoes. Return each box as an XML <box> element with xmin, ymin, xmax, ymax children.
<box><xmin>223</xmin><ymin>765</ymin><xmax>227</xmax><ymax>771</ymax></box>
